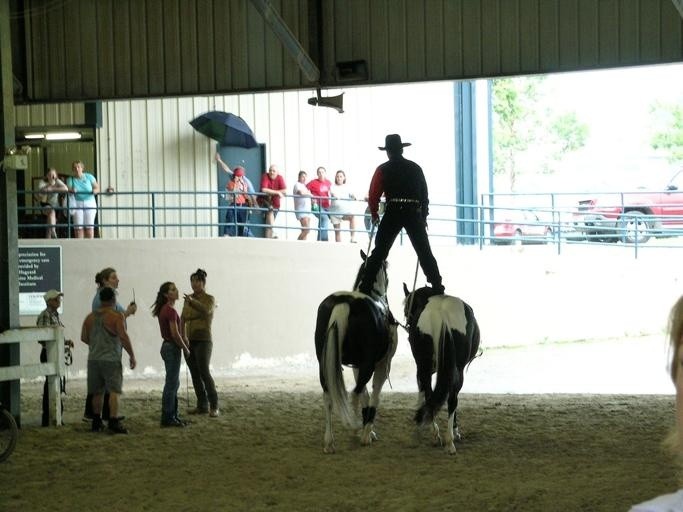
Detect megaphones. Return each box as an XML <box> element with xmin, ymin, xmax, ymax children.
<box><xmin>306</xmin><ymin>92</ymin><xmax>345</xmax><ymax>114</ymax></box>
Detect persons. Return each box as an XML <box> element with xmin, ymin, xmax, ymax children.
<box><xmin>178</xmin><ymin>268</ymin><xmax>219</xmax><ymax>418</ymax></box>
<box><xmin>357</xmin><ymin>134</ymin><xmax>446</xmax><ymax>296</ymax></box>
<box><xmin>293</xmin><ymin>170</ymin><xmax>318</xmax><ymax>241</ymax></box>
<box><xmin>66</xmin><ymin>161</ymin><xmax>100</xmax><ymax>238</ymax></box>
<box><xmin>363</xmin><ymin>190</ymin><xmax>388</xmax><ymax>240</ymax></box>
<box><xmin>81</xmin><ymin>286</ymin><xmax>137</xmax><ymax>434</ymax></box>
<box><xmin>35</xmin><ymin>288</ymin><xmax>75</xmax><ymax>427</ymax></box>
<box><xmin>215</xmin><ymin>154</ymin><xmax>259</xmax><ymax>237</ymax></box>
<box><xmin>306</xmin><ymin>166</ymin><xmax>333</xmax><ymax>241</ymax></box>
<box><xmin>81</xmin><ymin>267</ymin><xmax>137</xmax><ymax>422</ymax></box>
<box><xmin>329</xmin><ymin>170</ymin><xmax>359</xmax><ymax>243</ymax></box>
<box><xmin>36</xmin><ymin>167</ymin><xmax>68</xmax><ymax>238</ymax></box>
<box><xmin>150</xmin><ymin>281</ymin><xmax>190</xmax><ymax>427</ymax></box>
<box><xmin>257</xmin><ymin>164</ymin><xmax>287</xmax><ymax>238</ymax></box>
<box><xmin>221</xmin><ymin>166</ymin><xmax>250</xmax><ymax>236</ymax></box>
<box><xmin>627</xmin><ymin>295</ymin><xmax>683</xmax><ymax>511</ymax></box>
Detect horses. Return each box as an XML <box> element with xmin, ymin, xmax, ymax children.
<box><xmin>313</xmin><ymin>246</ymin><xmax>399</xmax><ymax>455</ymax></box>
<box><xmin>401</xmin><ymin>281</ymin><xmax>484</xmax><ymax>460</ymax></box>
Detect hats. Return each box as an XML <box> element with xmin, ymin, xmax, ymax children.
<box><xmin>378</xmin><ymin>134</ymin><xmax>411</xmax><ymax>150</ymax></box>
<box><xmin>44</xmin><ymin>289</ymin><xmax>64</xmax><ymax>302</ymax></box>
<box><xmin>234</xmin><ymin>168</ymin><xmax>244</xmax><ymax>176</ymax></box>
<box><xmin>100</xmin><ymin>287</ymin><xmax>118</xmax><ymax>300</ymax></box>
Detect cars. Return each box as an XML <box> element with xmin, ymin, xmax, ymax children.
<box><xmin>491</xmin><ymin>204</ymin><xmax>554</xmax><ymax>244</ymax></box>
<box><xmin>572</xmin><ymin>170</ymin><xmax>682</xmax><ymax>243</ymax></box>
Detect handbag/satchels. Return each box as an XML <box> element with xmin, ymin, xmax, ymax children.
<box><xmin>62</xmin><ymin>192</ymin><xmax>77</xmax><ymax>216</ymax></box>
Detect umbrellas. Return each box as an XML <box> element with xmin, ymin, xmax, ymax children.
<box><xmin>189</xmin><ymin>109</ymin><xmax>259</xmax><ymax>153</ymax></box>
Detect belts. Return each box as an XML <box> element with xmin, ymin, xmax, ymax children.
<box><xmin>389</xmin><ymin>199</ymin><xmax>420</xmax><ymax>204</ymax></box>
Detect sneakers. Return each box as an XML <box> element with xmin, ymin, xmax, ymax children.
<box><xmin>83</xmin><ymin>415</ymin><xmax>93</xmax><ymax>422</ymax></box>
<box><xmin>92</xmin><ymin>419</ymin><xmax>106</xmax><ymax>431</ymax></box>
<box><xmin>186</xmin><ymin>408</ymin><xmax>209</xmax><ymax>414</ymax></box>
<box><xmin>107</xmin><ymin>423</ymin><xmax>128</xmax><ymax>434</ymax></box>
<box><xmin>210</xmin><ymin>408</ymin><xmax>219</xmax><ymax>417</ymax></box>
<box><xmin>160</xmin><ymin>417</ymin><xmax>185</xmax><ymax>428</ymax></box>
